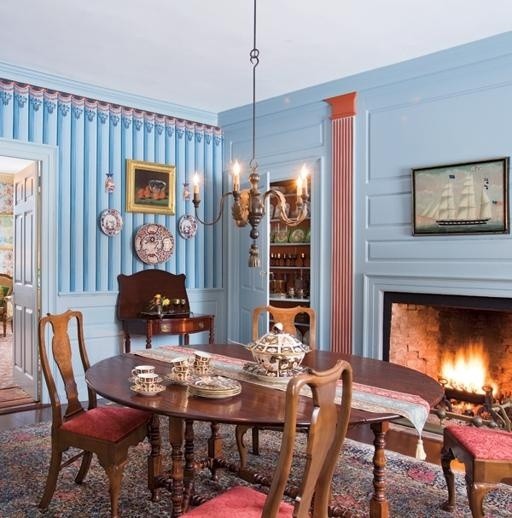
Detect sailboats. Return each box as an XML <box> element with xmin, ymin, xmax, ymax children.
<box><xmin>419</xmin><ymin>169</ymin><xmax>497</xmax><ymax>226</ymax></box>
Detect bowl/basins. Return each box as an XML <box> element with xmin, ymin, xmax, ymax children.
<box><xmin>246</xmin><ymin>323</ymin><xmax>313</xmax><ymax>370</ymax></box>
<box><xmin>148</xmin><ymin>180</ymin><xmax>167</xmax><ymax>193</ymax></box>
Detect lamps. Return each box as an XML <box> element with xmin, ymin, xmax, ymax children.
<box><xmin>191</xmin><ymin>0</ymin><xmax>312</xmax><ymax>269</ymax></box>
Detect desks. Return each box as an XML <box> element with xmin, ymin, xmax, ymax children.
<box><xmin>121</xmin><ymin>315</ymin><xmax>219</xmax><ymax>354</ymax></box>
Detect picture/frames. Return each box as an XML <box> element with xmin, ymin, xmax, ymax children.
<box><xmin>409</xmin><ymin>154</ymin><xmax>511</xmax><ymax>237</ymax></box>
<box><xmin>124</xmin><ymin>158</ymin><xmax>178</xmax><ymax>216</ymax></box>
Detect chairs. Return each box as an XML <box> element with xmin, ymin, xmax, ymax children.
<box><xmin>439</xmin><ymin>417</ymin><xmax>511</xmax><ymax>517</ymax></box>
<box><xmin>250</xmin><ymin>304</ymin><xmax>317</xmax><ymax>345</ymax></box>
<box><xmin>174</xmin><ymin>356</ymin><xmax>356</xmax><ymax>517</ymax></box>
<box><xmin>32</xmin><ymin>307</ymin><xmax>159</xmax><ymax>518</ymax></box>
<box><xmin>0</xmin><ymin>272</ymin><xmax>14</xmax><ymax>338</ymax></box>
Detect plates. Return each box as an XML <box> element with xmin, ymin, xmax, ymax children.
<box><xmin>98</xmin><ymin>209</ymin><xmax>123</xmax><ymax>236</ymax></box>
<box><xmin>188</xmin><ymin>377</ymin><xmax>245</xmax><ymax>400</ymax></box>
<box><xmin>126</xmin><ymin>375</ymin><xmax>164</xmax><ymax>384</ymax></box>
<box><xmin>271</xmin><ymin>229</ymin><xmax>310</xmax><ymax>243</ymax></box>
<box><xmin>134</xmin><ymin>223</ymin><xmax>175</xmax><ymax>265</ymax></box>
<box><xmin>270</xmin><ymin>201</ymin><xmax>310</xmax><ymax>219</ymax></box>
<box><xmin>241</xmin><ymin>361</ymin><xmax>317</xmax><ymax>385</ymax></box>
<box><xmin>130</xmin><ymin>385</ymin><xmax>167</xmax><ymax>396</ymax></box>
<box><xmin>178</xmin><ymin>215</ymin><xmax>198</xmax><ymax>240</ymax></box>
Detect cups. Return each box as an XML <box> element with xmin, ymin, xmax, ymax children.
<box><xmin>131</xmin><ymin>365</ymin><xmax>155</xmax><ymax>378</ymax></box>
<box><xmin>288</xmin><ymin>288</ymin><xmax>307</xmax><ymax>298</ymax></box>
<box><xmin>134</xmin><ymin>373</ymin><xmax>157</xmax><ymax>391</ymax></box>
<box><xmin>170</xmin><ymin>350</ymin><xmax>210</xmax><ymax>386</ymax></box>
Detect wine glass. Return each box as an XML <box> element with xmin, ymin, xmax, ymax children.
<box><xmin>270</xmin><ymin>252</ymin><xmax>306</xmax><ymax>267</ymax></box>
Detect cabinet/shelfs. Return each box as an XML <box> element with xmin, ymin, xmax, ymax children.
<box><xmin>267</xmin><ymin>174</ymin><xmax>312</xmax><ymax>334</ymax></box>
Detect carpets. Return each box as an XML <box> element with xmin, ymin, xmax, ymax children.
<box><xmin>1</xmin><ymin>422</ymin><xmax>512</xmax><ymax>518</ymax></box>
<box><xmin>1</xmin><ymin>401</ymin><xmax>511</xmax><ymax>518</ymax></box>
